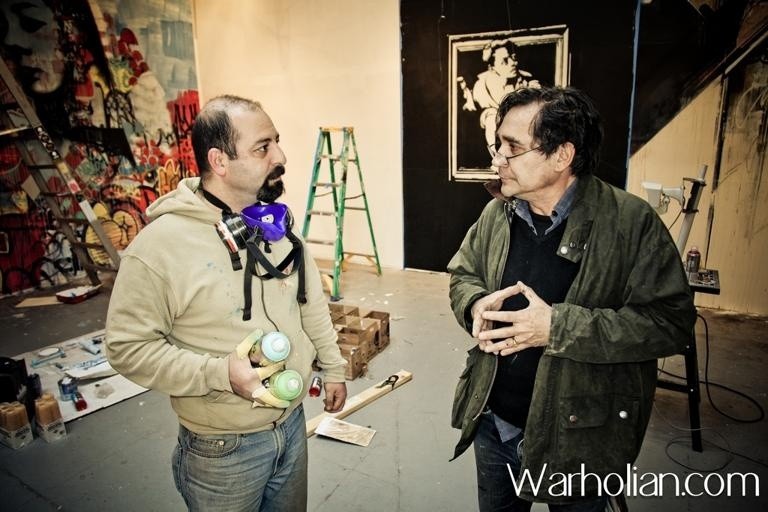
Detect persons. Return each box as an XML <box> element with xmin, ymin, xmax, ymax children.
<box><xmin>446</xmin><ymin>86</ymin><xmax>697</xmax><ymax>511</ymax></box>
<box><xmin>472</xmin><ymin>39</ymin><xmax>542</xmax><ymax>152</ymax></box>
<box><xmin>105</xmin><ymin>95</ymin><xmax>347</xmax><ymax>512</ymax></box>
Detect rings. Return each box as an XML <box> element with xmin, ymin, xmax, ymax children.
<box><xmin>512</xmin><ymin>337</ymin><xmax>518</xmax><ymax>347</ymax></box>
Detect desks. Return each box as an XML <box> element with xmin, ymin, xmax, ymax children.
<box><xmin>656</xmin><ymin>269</ymin><xmax>720</xmax><ymax>452</ymax></box>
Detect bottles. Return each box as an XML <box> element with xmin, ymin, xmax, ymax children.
<box><xmin>262</xmin><ymin>370</ymin><xmax>304</xmax><ymax>400</ymax></box>
<box><xmin>247</xmin><ymin>332</ymin><xmax>290</xmax><ymax>368</ymax></box>
<box><xmin>70</xmin><ymin>389</ymin><xmax>87</xmax><ymax>411</ymax></box>
<box><xmin>58</xmin><ymin>378</ymin><xmax>79</xmax><ymax>401</ymax></box>
<box><xmin>310</xmin><ymin>377</ymin><xmax>321</xmax><ymax>397</ymax></box>
<box><xmin>686</xmin><ymin>246</ymin><xmax>700</xmax><ymax>274</ymax></box>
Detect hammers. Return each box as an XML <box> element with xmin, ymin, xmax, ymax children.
<box><xmin>32</xmin><ymin>347</ymin><xmax>65</xmax><ymax>365</ymax></box>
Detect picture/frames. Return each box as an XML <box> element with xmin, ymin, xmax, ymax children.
<box><xmin>446</xmin><ymin>24</ymin><xmax>571</xmax><ymax>183</ymax></box>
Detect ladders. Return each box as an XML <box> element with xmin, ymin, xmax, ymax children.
<box><xmin>302</xmin><ymin>126</ymin><xmax>381</xmax><ymax>302</ymax></box>
<box><xmin>0</xmin><ymin>56</ymin><xmax>122</xmax><ymax>286</ymax></box>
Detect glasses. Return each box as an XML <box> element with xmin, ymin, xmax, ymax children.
<box><xmin>487</xmin><ymin>141</ymin><xmax>552</xmax><ymax>168</ymax></box>
